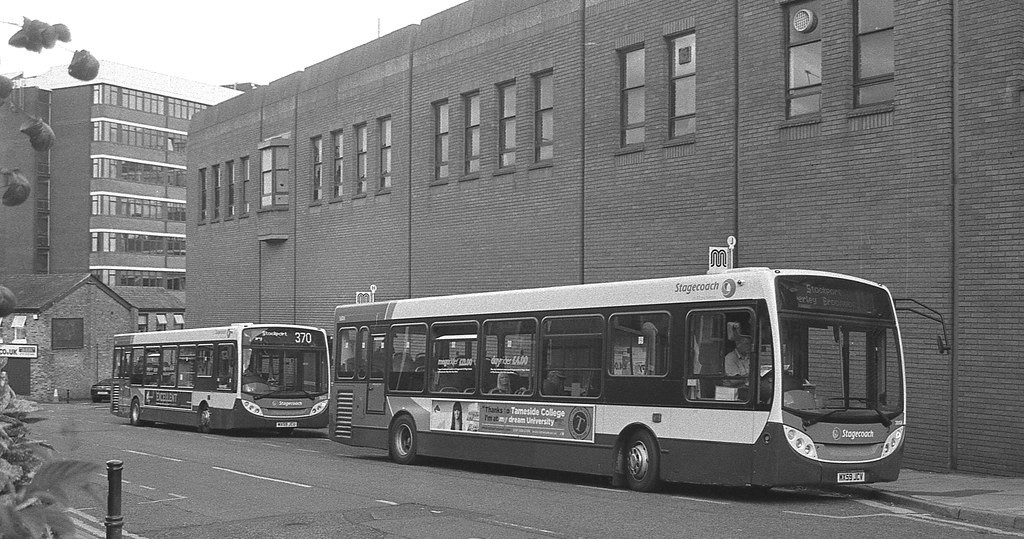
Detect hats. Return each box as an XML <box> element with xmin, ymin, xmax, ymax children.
<box><xmin>549</xmin><ymin>371</ymin><xmax>566</xmax><ymax>379</ymax></box>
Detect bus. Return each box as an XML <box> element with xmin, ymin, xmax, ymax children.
<box><xmin>108</xmin><ymin>321</ymin><xmax>332</xmax><ymax>437</ymax></box>
<box><xmin>325</xmin><ymin>235</ymin><xmax>952</xmax><ymax>497</ymax></box>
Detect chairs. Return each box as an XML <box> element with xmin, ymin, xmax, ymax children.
<box><xmin>440</xmin><ymin>387</ymin><xmax>459</xmax><ymax>392</ymax></box>
<box><xmin>463</xmin><ymin>388</ymin><xmax>475</xmax><ymax>393</ymax></box>
<box><xmin>414</xmin><ymin>353</ymin><xmax>426</xmax><ymax>372</ymax></box>
<box><xmin>392</xmin><ymin>351</ymin><xmax>416</xmax><ymax>372</ymax></box>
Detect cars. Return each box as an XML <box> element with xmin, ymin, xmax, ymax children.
<box><xmin>91</xmin><ymin>378</ymin><xmax>112</xmax><ymax>402</ymax></box>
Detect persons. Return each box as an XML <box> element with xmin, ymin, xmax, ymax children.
<box><xmin>497</xmin><ymin>372</ymin><xmax>526</xmax><ymax>395</ymax></box>
<box><xmin>542</xmin><ymin>370</ymin><xmax>567</xmax><ymax>396</ymax></box>
<box><xmin>724</xmin><ymin>335</ymin><xmax>753</xmax><ymax>400</ymax></box>
